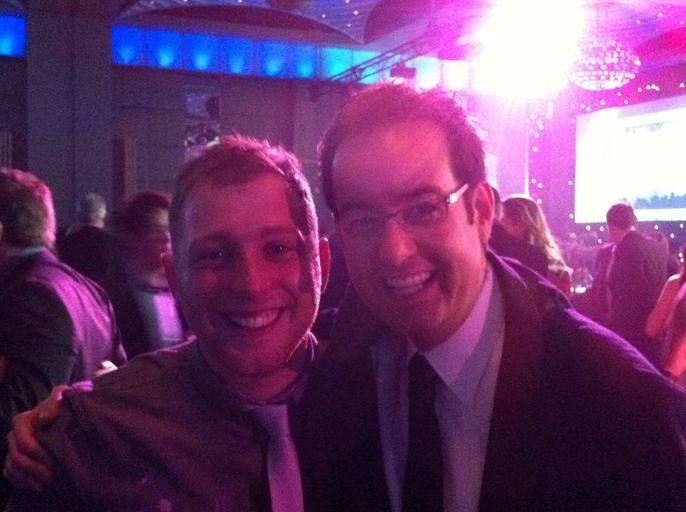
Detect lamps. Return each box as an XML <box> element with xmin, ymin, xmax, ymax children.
<box><xmin>571</xmin><ymin>3</ymin><xmax>640</xmax><ymax>91</ymax></box>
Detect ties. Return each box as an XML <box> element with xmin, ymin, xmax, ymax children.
<box><xmin>398</xmin><ymin>350</ymin><xmax>447</xmax><ymax>512</ymax></box>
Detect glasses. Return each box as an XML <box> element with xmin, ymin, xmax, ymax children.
<box><xmin>339</xmin><ymin>184</ymin><xmax>471</xmax><ymax>239</ymax></box>
